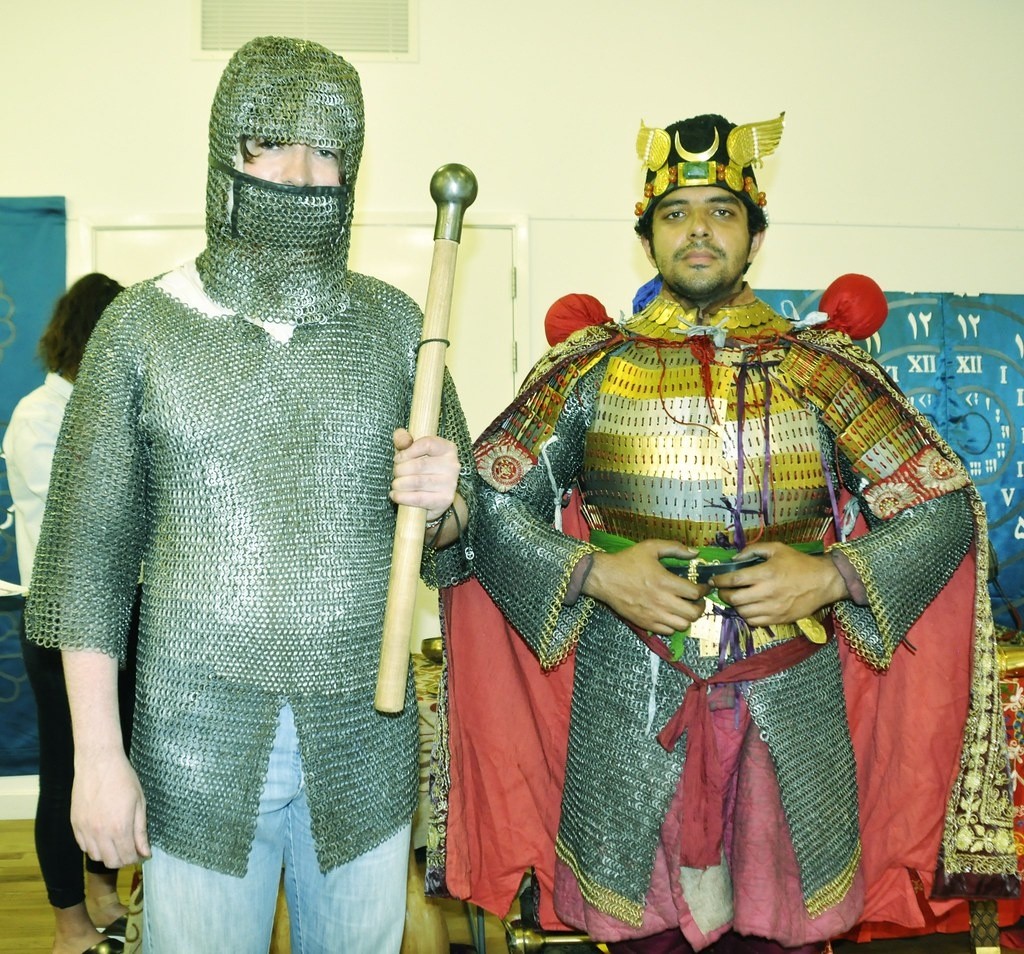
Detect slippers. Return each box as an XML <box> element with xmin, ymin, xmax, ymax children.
<box><xmin>41</xmin><ymin>911</ymin><xmax>127</xmax><ymax>954</ymax></box>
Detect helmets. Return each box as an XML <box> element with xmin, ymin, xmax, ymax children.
<box><xmin>195</xmin><ymin>34</ymin><xmax>368</xmax><ymax>324</ymax></box>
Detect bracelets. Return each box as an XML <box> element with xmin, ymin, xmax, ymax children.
<box><xmin>424</xmin><ymin>509</ymin><xmax>452</xmax><ymax>529</ymax></box>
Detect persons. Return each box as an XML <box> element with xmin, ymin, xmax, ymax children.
<box><xmin>470</xmin><ymin>115</ymin><xmax>973</xmax><ymax>954</ymax></box>
<box><xmin>26</xmin><ymin>35</ymin><xmax>472</xmax><ymax>954</ymax></box>
<box><xmin>3</xmin><ymin>273</ymin><xmax>144</xmax><ymax>954</ymax></box>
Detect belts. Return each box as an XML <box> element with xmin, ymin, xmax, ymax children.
<box><xmin>661</xmin><ymin>555</ymin><xmax>772</xmax><ymax>589</ymax></box>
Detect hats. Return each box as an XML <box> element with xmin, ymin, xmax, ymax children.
<box><xmin>631</xmin><ymin>112</ymin><xmax>782</xmax><ymax>213</ymax></box>
